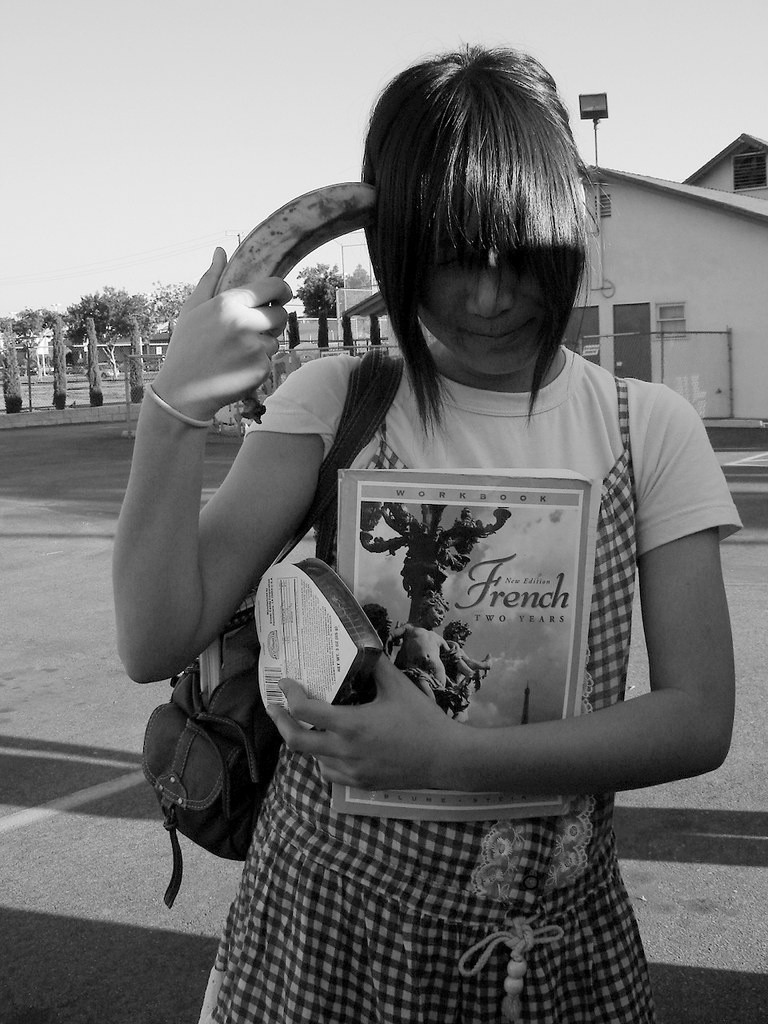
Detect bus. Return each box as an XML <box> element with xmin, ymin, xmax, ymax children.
<box><xmin>127</xmin><ymin>354</ymin><xmax>162</xmax><ymax>372</ymax></box>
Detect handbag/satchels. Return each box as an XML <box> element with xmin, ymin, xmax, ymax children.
<box><xmin>141</xmin><ymin>348</ymin><xmax>404</xmax><ymax>909</ymax></box>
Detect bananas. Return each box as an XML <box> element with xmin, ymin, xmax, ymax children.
<box><xmin>211</xmin><ymin>182</ymin><xmax>377</xmax><ymax>423</ymax></box>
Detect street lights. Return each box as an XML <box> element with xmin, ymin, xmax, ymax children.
<box><xmin>19</xmin><ymin>332</ymin><xmax>38</xmax><ymax>411</ymax></box>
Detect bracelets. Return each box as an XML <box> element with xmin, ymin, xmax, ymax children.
<box><xmin>144</xmin><ymin>381</ymin><xmax>216</xmax><ymax>428</ymax></box>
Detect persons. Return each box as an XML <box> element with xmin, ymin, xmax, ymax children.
<box><xmin>114</xmin><ymin>45</ymin><xmax>742</xmax><ymax>1024</ymax></box>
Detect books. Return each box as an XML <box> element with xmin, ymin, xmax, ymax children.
<box><xmin>335</xmin><ymin>467</ymin><xmax>590</xmax><ymax>823</ymax></box>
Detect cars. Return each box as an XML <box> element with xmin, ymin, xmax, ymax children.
<box><xmin>98</xmin><ymin>363</ymin><xmax>119</xmax><ymax>378</ymax></box>
